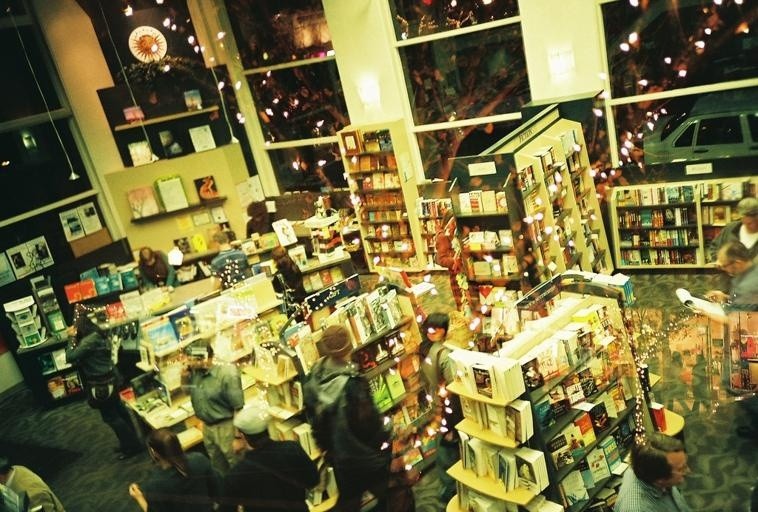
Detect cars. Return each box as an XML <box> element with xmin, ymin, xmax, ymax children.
<box><xmin>641</xmin><ymin>87</ymin><xmax>758</xmax><ymax>165</ymax></box>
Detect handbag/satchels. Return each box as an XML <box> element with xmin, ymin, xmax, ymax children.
<box><xmin>83</xmin><ymin>374</ymin><xmax>120</xmax><ymax>410</ymax></box>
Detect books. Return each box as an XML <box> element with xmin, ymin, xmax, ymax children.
<box><xmin>125</xmin><ymin>185</ymin><xmax>159</xmax><ymax>219</ymax></box>
<box><xmin>127</xmin><ymin>139</ymin><xmax>153</xmax><ymax>167</ymax></box>
<box><xmin>158</xmin><ymin>129</ymin><xmax>176</xmax><ymax>159</ymax></box>
<box><xmin>122</xmin><ymin>106</ymin><xmax>145</xmax><ymax>125</ymax></box>
<box><xmin>33</xmin><ymin>344</ymin><xmax>86</xmax><ymax>403</ymax></box>
<box><xmin>336</xmin><ymin>118</ymin><xmax>614</xmax><ymax>281</ymax></box>
<box><xmin>188</xmin><ymin>124</ymin><xmax>216</xmax><ymax>153</ymax></box>
<box><xmin>0</xmin><ymin>201</ymin><xmax>104</xmax><ymax>288</ymax></box>
<box><xmin>141</xmin><ymin>273</ymin><xmax>641</xmax><ymax>511</ymax></box>
<box><xmin>613</xmin><ymin>181</ymin><xmax>757</xmax><ymax>267</ymax></box>
<box><xmin>674</xmin><ymin>287</ymin><xmax>729</xmax><ymax>325</ymax></box>
<box><xmin>648</xmin><ymin>402</ymin><xmax>667</xmax><ymax>432</ymax></box>
<box><xmin>183</xmin><ymin>89</ymin><xmax>203</xmax><ymax>111</ymax></box>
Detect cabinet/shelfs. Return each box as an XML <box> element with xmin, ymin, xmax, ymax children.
<box><xmin>448</xmin><ymin>108</ymin><xmax>614</xmax><ymax>274</ymax></box>
<box><xmin>2</xmin><ymin>189</ymin><xmax>132</xmax><ymax>410</ymax></box>
<box><xmin>338</xmin><ymin>120</ymin><xmax>458</xmax><ymax>275</ymax></box>
<box><xmin>441</xmin><ymin>273</ymin><xmax>684</xmax><ymax>512</ymax></box>
<box><xmin>95</xmin><ymin>278</ymin><xmax>292</xmax><ymax>454</ymax></box>
<box><xmin>128</xmin><ymin>177</ymin><xmax>362</xmax><ymax>273</ymax></box>
<box><xmin>247</xmin><ymin>273</ymin><xmax>440</xmax><ymax>512</ymax></box>
<box><xmin>606</xmin><ymin>175</ymin><xmax>757</xmax><ymax>269</ymax></box>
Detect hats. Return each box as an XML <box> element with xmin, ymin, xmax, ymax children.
<box><xmin>231</xmin><ymin>405</ymin><xmax>272</xmax><ymax>449</ymax></box>
<box><xmin>319</xmin><ymin>324</ymin><xmax>353</xmax><ymax>357</ymax></box>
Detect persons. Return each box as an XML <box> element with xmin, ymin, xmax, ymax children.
<box><xmin>0</xmin><ymin>455</ymin><xmax>67</xmax><ymax>512</ymax></box>
<box><xmin>690</xmin><ymin>239</ymin><xmax>757</xmax><ymax>323</ymax></box>
<box><xmin>63</xmin><ymin>314</ymin><xmax>142</xmax><ymax>461</ymax></box>
<box><xmin>207</xmin><ymin>231</ymin><xmax>253</xmax><ymax>292</ymax></box>
<box><xmin>129</xmin><ymin>429</ymin><xmax>217</xmax><ymax>510</ymax></box>
<box><xmin>245</xmin><ymin>199</ymin><xmax>279</xmax><ymax>240</ymax></box>
<box><xmin>642</xmin><ymin>341</ymin><xmax>717</xmax><ymax>423</ymax></box>
<box><xmin>137</xmin><ymin>245</ymin><xmax>178</xmax><ymax>295</ymax></box>
<box><xmin>608</xmin><ymin>431</ymin><xmax>694</xmax><ymax>511</ymax></box>
<box><xmin>271</xmin><ymin>245</ymin><xmax>309</xmax><ymax>323</ymax></box>
<box><xmin>712</xmin><ymin>195</ymin><xmax>758</xmax><ymax>294</ymax></box>
